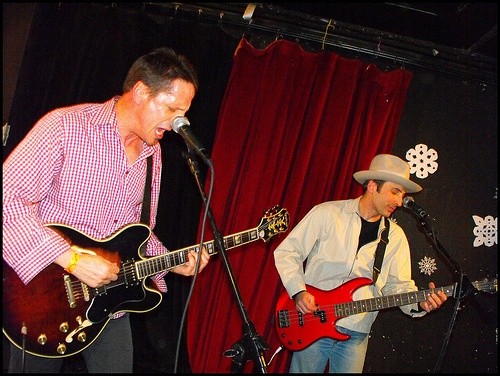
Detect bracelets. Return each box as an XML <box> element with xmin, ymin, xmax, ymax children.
<box><xmin>65</xmin><ymin>251</ymin><xmax>80</xmax><ymax>273</ymax></box>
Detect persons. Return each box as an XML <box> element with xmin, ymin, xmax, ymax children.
<box><xmin>273</xmin><ymin>154</ymin><xmax>447</xmax><ymax>374</ymax></box>
<box><xmin>3</xmin><ymin>48</ymin><xmax>210</xmax><ymax>372</ymax></box>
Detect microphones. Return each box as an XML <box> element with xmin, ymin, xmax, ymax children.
<box><xmin>171</xmin><ymin>116</ymin><xmax>211</xmax><ymax>166</ymax></box>
<box><xmin>401</xmin><ymin>196</ymin><xmax>426</xmax><ymax>217</ymax></box>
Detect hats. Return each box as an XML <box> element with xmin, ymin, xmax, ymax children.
<box><xmin>353</xmin><ymin>153</ymin><xmax>423</xmax><ymax>194</ymax></box>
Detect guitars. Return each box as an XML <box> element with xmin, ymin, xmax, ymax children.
<box><xmin>0</xmin><ymin>205</ymin><xmax>290</xmax><ymax>358</ymax></box>
<box><xmin>273</xmin><ymin>277</ymin><xmax>498</xmax><ymax>352</ymax></box>
